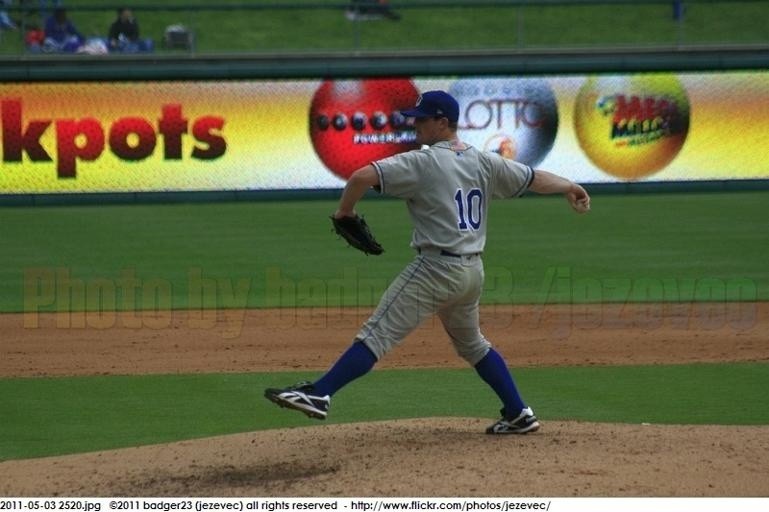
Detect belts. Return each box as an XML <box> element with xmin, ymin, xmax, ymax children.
<box><xmin>418</xmin><ymin>248</ymin><xmax>461</xmax><ymax>257</ymax></box>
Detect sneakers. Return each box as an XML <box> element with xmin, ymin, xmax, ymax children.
<box><xmin>486</xmin><ymin>406</ymin><xmax>540</xmax><ymax>434</ymax></box>
<box><xmin>265</xmin><ymin>382</ymin><xmax>331</xmax><ymax>420</ymax></box>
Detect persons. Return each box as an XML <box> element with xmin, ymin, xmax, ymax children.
<box><xmin>263</xmin><ymin>89</ymin><xmax>592</xmax><ymax>437</ymax></box>
<box><xmin>43</xmin><ymin>7</ymin><xmax>87</xmax><ymax>54</ymax></box>
<box><xmin>106</xmin><ymin>5</ymin><xmax>153</xmax><ymax>54</ymax></box>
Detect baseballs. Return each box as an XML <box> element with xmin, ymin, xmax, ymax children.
<box><xmin>574</xmin><ymin>72</ymin><xmax>689</xmax><ymax>179</ymax></box>
<box><xmin>575</xmin><ymin>201</ymin><xmax>588</xmax><ymax>211</ymax></box>
<box><xmin>309</xmin><ymin>79</ymin><xmax>420</xmax><ymax>182</ymax></box>
<box><xmin>446</xmin><ymin>74</ymin><xmax>558</xmax><ymax>168</ymax></box>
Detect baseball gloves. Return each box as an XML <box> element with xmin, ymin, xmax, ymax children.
<box><xmin>330</xmin><ymin>211</ymin><xmax>385</xmax><ymax>256</ymax></box>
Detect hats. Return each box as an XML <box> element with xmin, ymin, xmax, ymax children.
<box><xmin>399</xmin><ymin>90</ymin><xmax>458</xmax><ymax>121</ymax></box>
